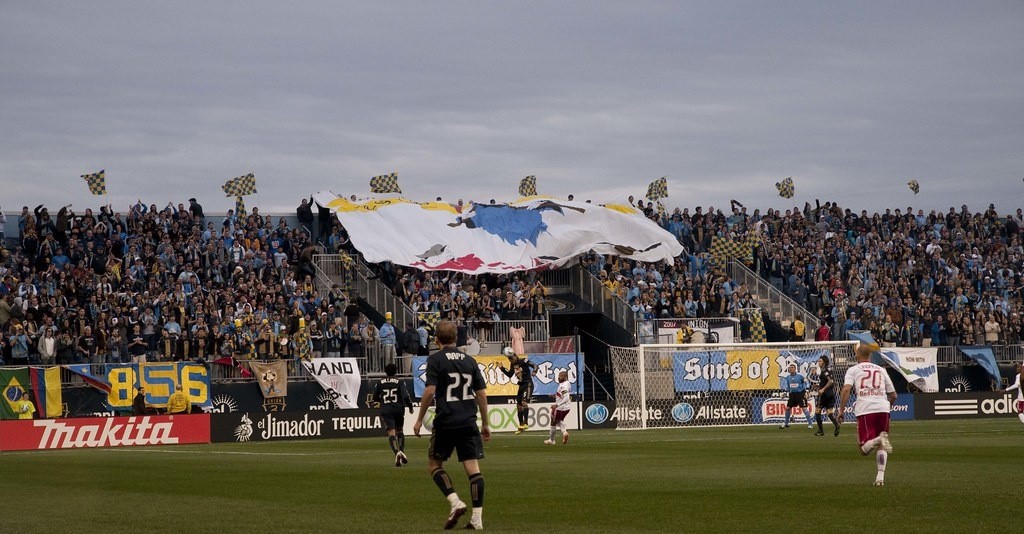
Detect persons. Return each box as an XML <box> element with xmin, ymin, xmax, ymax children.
<box><xmin>133</xmin><ymin>386</ymin><xmax>147</xmax><ymax>415</ymax></box>
<box><xmin>373</xmin><ymin>363</ymin><xmax>414</xmax><ymax>468</ymax></box>
<box><xmin>413</xmin><ymin>320</ymin><xmax>492</xmax><ymax>530</ymax></box>
<box><xmin>166</xmin><ymin>384</ymin><xmax>192</xmax><ymax>414</ymax></box>
<box><xmin>779</xmin><ymin>364</ymin><xmax>813</xmax><ymax>429</ymax></box>
<box><xmin>815</xmin><ymin>356</ymin><xmax>840</xmax><ymax>437</ymax></box>
<box><xmin>1000</xmin><ymin>361</ymin><xmax>1024</xmax><ymax>423</ymax></box>
<box><xmin>806</xmin><ymin>361</ymin><xmax>821</xmax><ymax>389</ymax></box>
<box><xmin>836</xmin><ymin>346</ymin><xmax>897</xmax><ymax>486</ymax></box>
<box><xmin>496</xmin><ymin>353</ymin><xmax>539</xmax><ymax>435</ymax></box>
<box><xmin>544</xmin><ymin>371</ymin><xmax>571</xmax><ymax>444</ymax></box>
<box><xmin>15</xmin><ymin>392</ymin><xmax>36</xmax><ymax>420</ymax></box>
<box><xmin>0</xmin><ymin>195</ymin><xmax>1024</xmax><ymax>389</ymax></box>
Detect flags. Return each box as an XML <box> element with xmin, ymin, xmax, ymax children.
<box><xmin>656</xmin><ymin>200</ymin><xmax>669</xmax><ymax>219</ymax></box>
<box><xmin>710</xmin><ymin>235</ymin><xmax>754</xmax><ymax>264</ymax></box>
<box><xmin>743</xmin><ymin>307</ymin><xmax>768</xmax><ymax>342</ymax></box>
<box><xmin>746</xmin><ymin>229</ymin><xmax>762</xmax><ymax>250</ymax></box>
<box><xmin>645</xmin><ymin>176</ymin><xmax>669</xmax><ymax>202</ymax></box>
<box><xmin>776</xmin><ymin>178</ymin><xmax>794</xmax><ymax>198</ymax></box>
<box><xmin>299</xmin><ymin>325</ymin><xmax>315</xmax><ymax>362</ymax></box>
<box><xmin>340</xmin><ymin>249</ymin><xmax>358</xmax><ymax>269</ymax></box>
<box><xmin>235</xmin><ymin>189</ymin><xmax>247</xmax><ymax>230</ymax></box>
<box><xmin>518</xmin><ymin>175</ymin><xmax>538</xmax><ymax>197</ymax></box>
<box><xmin>80</xmin><ymin>170</ymin><xmax>107</xmax><ymax>195</ymax></box>
<box><xmin>345</xmin><ymin>262</ymin><xmax>354</xmax><ymax>301</ymax></box>
<box><xmin>908</xmin><ymin>180</ymin><xmax>920</xmax><ymax>194</ymax></box>
<box><xmin>370</xmin><ymin>171</ymin><xmax>402</xmax><ymax>194</ymax></box>
<box><xmin>416</xmin><ymin>312</ymin><xmax>442</xmax><ymax>350</ymax></box>
<box><xmin>222</xmin><ymin>172</ymin><xmax>258</xmax><ymax>197</ymax></box>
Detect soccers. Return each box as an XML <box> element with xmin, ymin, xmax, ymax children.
<box><xmin>504</xmin><ymin>346</ymin><xmax>514</xmax><ymax>357</ymax></box>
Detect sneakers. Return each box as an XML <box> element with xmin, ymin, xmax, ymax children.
<box><xmin>444</xmin><ymin>501</ymin><xmax>467</xmax><ymax>529</ymax></box>
<box><xmin>515</xmin><ymin>429</ymin><xmax>523</xmax><ymax>435</ymax></box>
<box><xmin>394</xmin><ymin>452</ymin><xmax>401</xmax><ymax>467</ymax></box>
<box><xmin>462</xmin><ymin>519</ymin><xmax>483</xmax><ymax>530</ymax></box>
<box><xmin>815</xmin><ymin>430</ymin><xmax>824</xmax><ymax>436</ymax></box>
<box><xmin>544</xmin><ymin>439</ymin><xmax>556</xmax><ymax>445</ymax></box>
<box><xmin>562</xmin><ymin>433</ymin><xmax>569</xmax><ymax>444</ymax></box>
<box><xmin>399</xmin><ymin>451</ymin><xmax>408</xmax><ymax>464</ymax></box>
<box><xmin>872</xmin><ymin>480</ymin><xmax>884</xmax><ymax>486</ymax></box>
<box><xmin>519</xmin><ymin>423</ymin><xmax>528</xmax><ymax>429</ymax></box>
<box><xmin>879</xmin><ymin>431</ymin><xmax>892</xmax><ymax>455</ymax></box>
<box><xmin>808</xmin><ymin>426</ymin><xmax>814</xmax><ymax>429</ymax></box>
<box><xmin>779</xmin><ymin>423</ymin><xmax>790</xmax><ymax>429</ymax></box>
<box><xmin>835</xmin><ymin>424</ymin><xmax>840</xmax><ymax>436</ymax></box>
